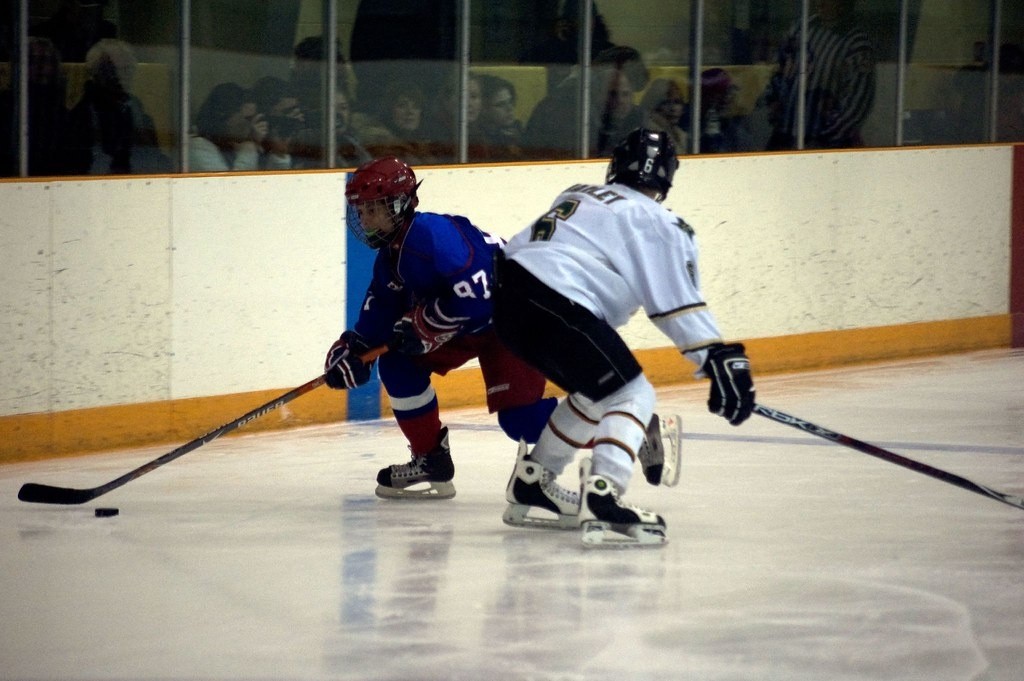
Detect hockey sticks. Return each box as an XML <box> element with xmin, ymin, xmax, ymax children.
<box><xmin>17</xmin><ymin>341</ymin><xmax>390</xmax><ymax>506</ymax></box>
<box><xmin>752</xmin><ymin>400</ymin><xmax>1023</xmax><ymax>511</ymax></box>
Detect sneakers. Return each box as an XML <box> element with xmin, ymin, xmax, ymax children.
<box><xmin>637</xmin><ymin>413</ymin><xmax>681</xmax><ymax>487</ymax></box>
<box><xmin>577</xmin><ymin>457</ymin><xmax>669</xmax><ymax>548</ymax></box>
<box><xmin>375</xmin><ymin>426</ymin><xmax>456</xmax><ymax>500</ymax></box>
<box><xmin>502</xmin><ymin>437</ymin><xmax>580</xmax><ymax>529</ymax></box>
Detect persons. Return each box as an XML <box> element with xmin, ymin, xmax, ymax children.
<box><xmin>490</xmin><ymin>129</ymin><xmax>757</xmax><ymax>533</ymax></box>
<box><xmin>327</xmin><ymin>159</ymin><xmax>667</xmax><ymax>492</ymax></box>
<box><xmin>0</xmin><ymin>1</ymin><xmax>992</xmax><ymax>175</ymax></box>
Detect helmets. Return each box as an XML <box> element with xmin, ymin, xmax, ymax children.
<box><xmin>608</xmin><ymin>128</ymin><xmax>679</xmax><ymax>201</ymax></box>
<box><xmin>345</xmin><ymin>157</ymin><xmax>419</xmax><ymax>209</ymax></box>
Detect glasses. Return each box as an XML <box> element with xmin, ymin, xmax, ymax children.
<box><xmin>491</xmin><ymin>97</ymin><xmax>514</xmax><ymax>107</ymax></box>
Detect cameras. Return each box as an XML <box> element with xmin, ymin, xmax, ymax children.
<box><xmin>255</xmin><ymin>111</ymin><xmax>301</xmax><ymax>141</ymax></box>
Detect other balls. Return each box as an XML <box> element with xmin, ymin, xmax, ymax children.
<box><xmin>95</xmin><ymin>508</ymin><xmax>120</xmax><ymax>518</ymax></box>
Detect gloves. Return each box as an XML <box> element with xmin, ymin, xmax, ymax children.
<box><xmin>394</xmin><ymin>304</ymin><xmax>461</xmax><ymax>355</ymax></box>
<box><xmin>325</xmin><ymin>330</ymin><xmax>377</xmax><ymax>389</ymax></box>
<box><xmin>703</xmin><ymin>343</ymin><xmax>755</xmax><ymax>425</ymax></box>
